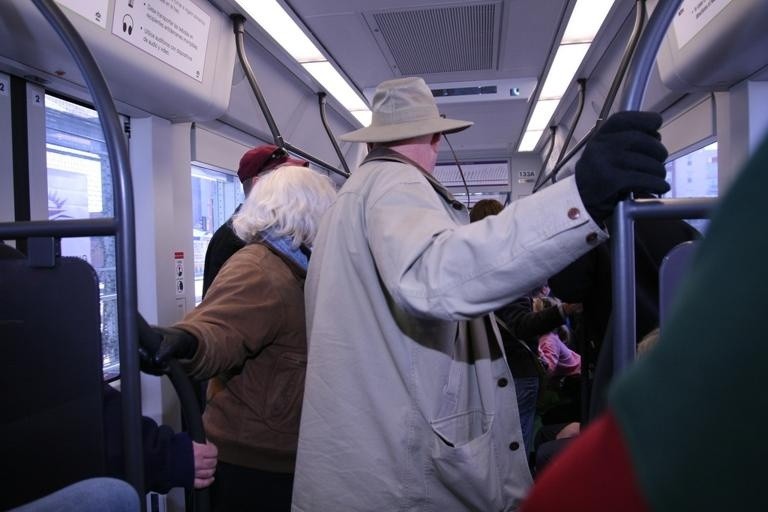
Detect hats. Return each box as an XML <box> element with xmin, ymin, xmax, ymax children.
<box><xmin>337</xmin><ymin>75</ymin><xmax>475</xmax><ymax>147</ymax></box>
<box><xmin>236</xmin><ymin>144</ymin><xmax>312</xmax><ymax>181</ymax></box>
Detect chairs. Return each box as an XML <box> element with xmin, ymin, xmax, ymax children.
<box><xmin>0</xmin><ymin>256</ymin><xmax>103</xmax><ymax>512</ymax></box>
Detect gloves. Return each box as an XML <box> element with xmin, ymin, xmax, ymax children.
<box><xmin>137</xmin><ymin>312</ymin><xmax>196</xmax><ymax>376</ymax></box>
<box><xmin>574</xmin><ymin>108</ymin><xmax>672</xmax><ymax>231</ymax></box>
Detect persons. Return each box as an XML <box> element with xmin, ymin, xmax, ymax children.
<box><xmin>2</xmin><ymin>75</ymin><xmax>767</xmax><ymax>512</ymax></box>
